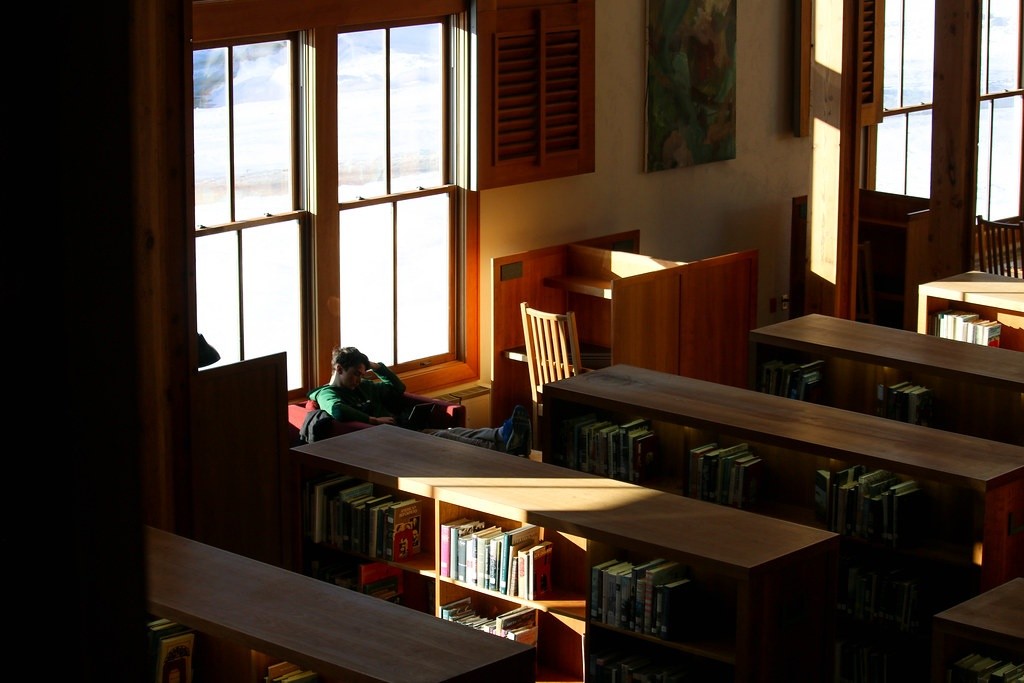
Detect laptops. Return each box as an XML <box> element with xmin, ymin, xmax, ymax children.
<box><xmin>392</xmin><ymin>402</ymin><xmax>435</xmax><ymax>428</ymax></box>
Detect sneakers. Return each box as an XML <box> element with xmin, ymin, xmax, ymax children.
<box><xmin>501</xmin><ymin>405</ymin><xmax>528</xmax><ymax>455</ymax></box>
<box><xmin>525</xmin><ymin>420</ymin><xmax>531</xmax><ymax>454</ymax></box>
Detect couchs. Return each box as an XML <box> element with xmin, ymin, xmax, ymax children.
<box><xmin>285</xmin><ymin>394</ymin><xmax>467</xmax><ymax>447</ymax></box>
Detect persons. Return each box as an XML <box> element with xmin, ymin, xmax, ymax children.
<box><xmin>307</xmin><ymin>346</ymin><xmax>532</xmax><ymax>454</ymax></box>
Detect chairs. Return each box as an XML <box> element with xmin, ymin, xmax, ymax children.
<box><xmin>856</xmin><ymin>241</ymin><xmax>876</xmax><ymax>323</ymax></box>
<box><xmin>975</xmin><ymin>215</ymin><xmax>1024</xmax><ymax>279</ymax></box>
<box><xmin>521</xmin><ymin>299</ymin><xmax>584</xmax><ymax>461</ymax></box>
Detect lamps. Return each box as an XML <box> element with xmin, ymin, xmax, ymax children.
<box><xmin>196</xmin><ymin>332</ymin><xmax>221</xmax><ymax>368</ymax></box>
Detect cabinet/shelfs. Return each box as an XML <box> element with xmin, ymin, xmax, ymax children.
<box><xmin>145</xmin><ymin>187</ymin><xmax>1024</xmax><ymax>683</ymax></box>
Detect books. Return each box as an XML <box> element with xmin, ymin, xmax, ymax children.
<box><xmin>551</xmin><ymin>415</ymin><xmax>658</xmax><ymax>483</ymax></box>
<box><xmin>814</xmin><ymin>469</ymin><xmax>831</xmax><ymax>524</ymax></box>
<box><xmin>302</xmin><ymin>472</ymin><xmax>422</xmax><ymax>563</ymax></box>
<box><xmin>948</xmin><ymin>652</ymin><xmax>1024</xmax><ymax>683</ymax></box>
<box><xmin>835</xmin><ymin>641</ymin><xmax>896</xmax><ymax>683</ymax></box>
<box><xmin>590</xmin><ymin>556</ymin><xmax>692</xmax><ymax>641</ymax></box>
<box><xmin>757</xmin><ymin>359</ymin><xmax>825</xmax><ymax>404</ymax></box>
<box><xmin>317</xmin><ymin>564</ymin><xmax>404</xmax><ymax>605</ymax></box>
<box><xmin>832</xmin><ymin>463</ymin><xmax>922</xmax><ymax>548</ymax></box>
<box><xmin>688</xmin><ymin>442</ymin><xmax>770</xmax><ymax>510</ymax></box>
<box><xmin>440</xmin><ymin>518</ymin><xmax>554</xmax><ymax>601</ymax></box>
<box><xmin>876</xmin><ymin>383</ymin><xmax>885</xmax><ymax>417</ymax></box>
<box><xmin>589</xmin><ymin>653</ymin><xmax>689</xmax><ymax>683</ymax></box>
<box><xmin>440</xmin><ymin>596</ymin><xmax>538</xmax><ymax>675</ymax></box>
<box><xmin>887</xmin><ymin>380</ymin><xmax>937</xmax><ymax>429</ymax></box>
<box><xmin>146</xmin><ymin>617</ymin><xmax>197</xmax><ymax>683</ymax></box>
<box><xmin>263</xmin><ymin>661</ymin><xmax>319</xmax><ymax>683</ymax></box>
<box><xmin>839</xmin><ymin>559</ymin><xmax>928</xmax><ymax>633</ymax></box>
<box><xmin>928</xmin><ymin>309</ymin><xmax>1002</xmax><ymax>348</ymax></box>
